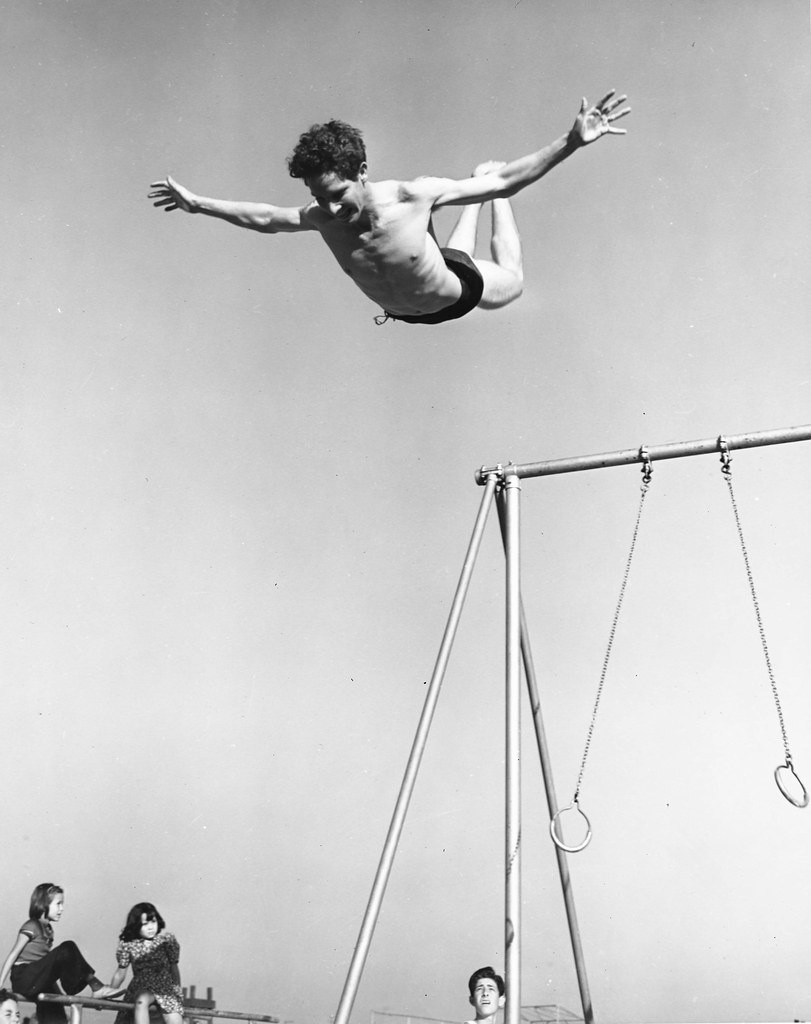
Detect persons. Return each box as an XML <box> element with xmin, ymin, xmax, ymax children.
<box><xmin>97</xmin><ymin>902</ymin><xmax>183</xmax><ymax>1024</ymax></box>
<box><xmin>464</xmin><ymin>967</ymin><xmax>506</xmax><ymax>1024</ymax></box>
<box><xmin>146</xmin><ymin>88</ymin><xmax>632</xmax><ymax>326</ymax></box>
<box><xmin>0</xmin><ymin>988</ymin><xmax>19</xmax><ymax>1023</ymax></box>
<box><xmin>0</xmin><ymin>883</ymin><xmax>128</xmax><ymax>1024</ymax></box>
